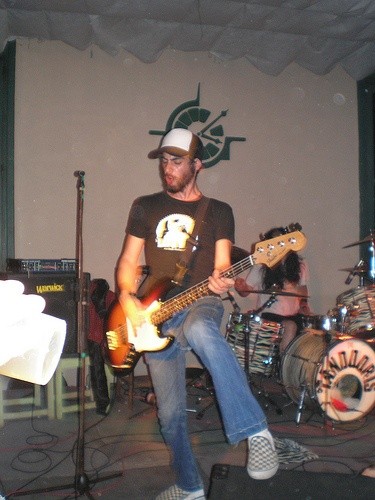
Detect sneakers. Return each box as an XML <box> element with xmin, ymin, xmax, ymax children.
<box><xmin>155</xmin><ymin>482</ymin><xmax>206</xmax><ymax>500</ymax></box>
<box><xmin>247</xmin><ymin>432</ymin><xmax>279</xmax><ymax>480</ymax></box>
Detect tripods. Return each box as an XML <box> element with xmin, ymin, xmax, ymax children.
<box><xmin>3</xmin><ymin>170</ymin><xmax>123</xmax><ymax>500</ymax></box>
<box><xmin>186</xmin><ymin>296</ymin><xmax>329</xmax><ymax>426</ymax></box>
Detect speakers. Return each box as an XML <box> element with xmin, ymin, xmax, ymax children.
<box><xmin>0</xmin><ymin>272</ymin><xmax>90</xmax><ymax>359</ymax></box>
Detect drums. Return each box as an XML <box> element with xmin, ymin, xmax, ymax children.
<box><xmin>336</xmin><ymin>283</ymin><xmax>375</xmax><ymax>343</ymax></box>
<box><xmin>225</xmin><ymin>311</ymin><xmax>286</xmax><ymax>378</ymax></box>
<box><xmin>279</xmin><ymin>327</ymin><xmax>375</xmax><ymax>422</ymax></box>
<box><xmin>303</xmin><ymin>315</ymin><xmax>336</xmax><ymax>331</ymax></box>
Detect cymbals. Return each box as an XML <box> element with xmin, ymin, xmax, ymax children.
<box><xmin>337</xmin><ymin>267</ymin><xmax>369</xmax><ymax>273</ymax></box>
<box><xmin>342</xmin><ymin>233</ymin><xmax>375</xmax><ymax>249</ymax></box>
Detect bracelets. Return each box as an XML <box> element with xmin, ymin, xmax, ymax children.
<box><xmin>300</xmin><ymin>299</ymin><xmax>308</xmax><ymax>304</ymax></box>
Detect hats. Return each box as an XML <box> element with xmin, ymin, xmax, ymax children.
<box><xmin>148</xmin><ymin>128</ymin><xmax>203</xmax><ymax>160</ymax></box>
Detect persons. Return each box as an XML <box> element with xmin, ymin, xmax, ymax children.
<box><xmin>235</xmin><ymin>228</ymin><xmax>312</xmax><ymax>364</ymax></box>
<box><xmin>115</xmin><ymin>128</ymin><xmax>279</xmax><ymax>499</ymax></box>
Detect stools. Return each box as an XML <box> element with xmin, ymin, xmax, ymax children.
<box><xmin>0</xmin><ymin>365</ymin><xmax>54</xmax><ymax>426</ymax></box>
<box><xmin>56</xmin><ymin>357</ymin><xmax>110</xmax><ymax>418</ymax></box>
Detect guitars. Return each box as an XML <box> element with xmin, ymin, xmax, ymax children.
<box><xmin>101</xmin><ymin>223</ymin><xmax>308</xmax><ymax>378</ymax></box>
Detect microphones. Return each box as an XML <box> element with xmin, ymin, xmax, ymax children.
<box><xmin>344</xmin><ymin>256</ymin><xmax>365</xmax><ymax>285</ymax></box>
<box><xmin>227</xmin><ymin>291</ymin><xmax>240</xmax><ymax>313</ymax></box>
